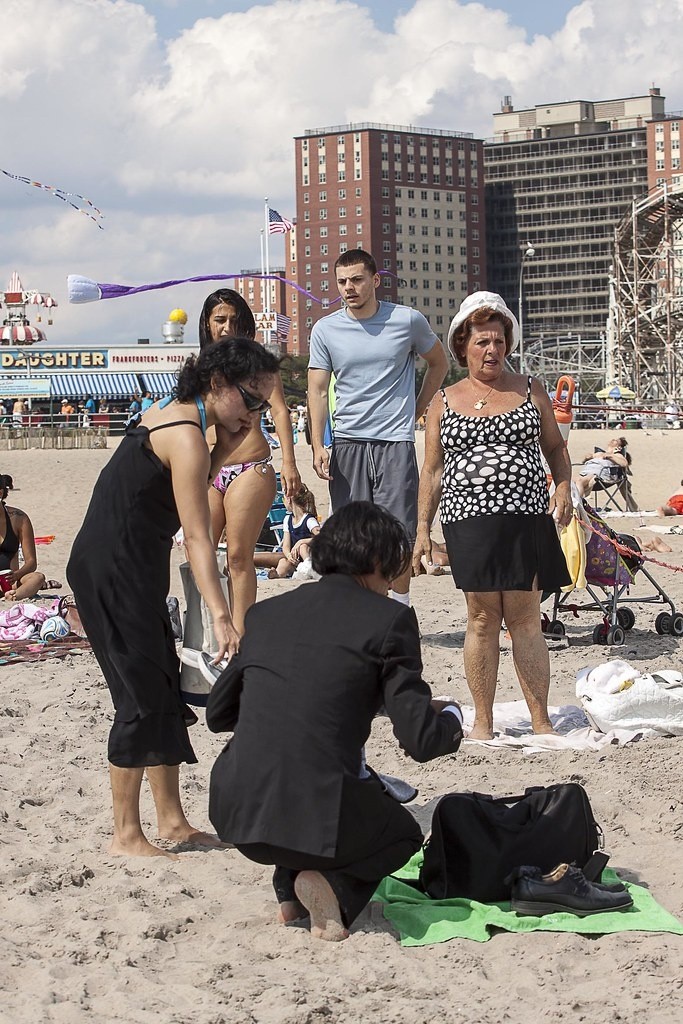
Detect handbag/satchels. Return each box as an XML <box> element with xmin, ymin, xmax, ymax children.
<box><xmin>576</xmin><ymin>662</ymin><xmax>682</xmax><ymax>732</ymax></box>
<box><xmin>178</xmin><ymin>562</ymin><xmax>230</xmax><ymax>707</ymax></box>
<box><xmin>421</xmin><ymin>783</ymin><xmax>603</xmax><ymax>901</ymax></box>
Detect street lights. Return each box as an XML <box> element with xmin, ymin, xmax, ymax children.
<box><xmin>520</xmin><ymin>249</ymin><xmax>535</xmax><ymax>374</ymax></box>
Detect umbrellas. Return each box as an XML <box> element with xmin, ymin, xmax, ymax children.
<box><xmin>596</xmin><ymin>385</ymin><xmax>636</xmax><ymax>409</ymax></box>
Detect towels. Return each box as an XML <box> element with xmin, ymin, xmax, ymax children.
<box><xmin>597</xmin><ymin>509</ymin><xmax>657</xmax><ymax>515</ymax></box>
<box><xmin>1</xmin><ymin>598</ymin><xmax>70</xmax><ymax>642</ymax></box>
<box><xmin>460</xmin><ymin>699</ymin><xmax>639</xmax><ymax>753</ymax></box>
<box><xmin>633</xmin><ymin>524</ymin><xmax>683</xmax><ymax>534</ymax></box>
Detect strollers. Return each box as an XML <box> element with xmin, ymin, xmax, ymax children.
<box><xmin>540</xmin><ymin>480</ymin><xmax>683</xmax><ymax>645</ymax></box>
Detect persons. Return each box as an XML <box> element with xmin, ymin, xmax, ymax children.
<box><xmin>658</xmin><ymin>479</ymin><xmax>683</xmax><ymax>518</ymax></box>
<box><xmin>253</xmin><ymin>481</ymin><xmax>322</xmax><ymax>579</ymax></box>
<box><xmin>205</xmin><ymin>498</ymin><xmax>464</xmax><ymax>942</ymax></box>
<box><xmin>411</xmin><ymin>290</ymin><xmax>574</xmax><ymax>742</ymax></box>
<box><xmin>133</xmin><ymin>386</ymin><xmax>162</xmax><ymax>411</ymax></box>
<box><xmin>0</xmin><ymin>395</ymin><xmax>139</xmax><ymax>431</ymax></box>
<box><xmin>0</xmin><ymin>473</ymin><xmax>46</xmax><ymax>604</ymax></box>
<box><xmin>306</xmin><ymin>250</ymin><xmax>449</xmax><ymax>609</ymax></box>
<box><xmin>199</xmin><ymin>289</ymin><xmax>301</xmax><ymax>641</ymax></box>
<box><xmin>570</xmin><ymin>436</ymin><xmax>630</xmax><ymax>497</ymax></box>
<box><xmin>66</xmin><ymin>335</ymin><xmax>282</xmax><ymax>866</ymax></box>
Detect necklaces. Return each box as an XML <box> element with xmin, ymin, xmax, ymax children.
<box><xmin>467</xmin><ymin>374</ymin><xmax>503</xmax><ymax>410</ymax></box>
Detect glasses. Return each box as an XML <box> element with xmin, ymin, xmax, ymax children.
<box><xmin>234</xmin><ymin>383</ymin><xmax>272</xmax><ymax>414</ymax></box>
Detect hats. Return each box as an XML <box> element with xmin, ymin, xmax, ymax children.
<box><xmin>61</xmin><ymin>399</ymin><xmax>68</xmax><ymax>404</ymax></box>
<box><xmin>447</xmin><ymin>290</ymin><xmax>519</xmax><ymax>365</ymax></box>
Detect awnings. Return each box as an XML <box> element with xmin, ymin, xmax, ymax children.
<box><xmin>1</xmin><ymin>374</ymin><xmax>142</xmax><ymax>399</ymax></box>
<box><xmin>142</xmin><ymin>372</ymin><xmax>181</xmax><ymax>398</ymax></box>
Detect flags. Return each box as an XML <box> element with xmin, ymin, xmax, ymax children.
<box><xmin>269</xmin><ymin>208</ymin><xmax>298</xmax><ymax>235</ymax></box>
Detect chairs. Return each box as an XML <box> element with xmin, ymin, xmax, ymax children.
<box><xmin>572</xmin><ymin>463</ymin><xmax>629</xmax><ymax>513</ymax></box>
<box><xmin>268</xmin><ymin>473</ymin><xmax>286</xmax><ymax>553</ymax></box>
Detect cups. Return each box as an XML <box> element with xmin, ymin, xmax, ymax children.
<box><xmin>0</xmin><ymin>569</ymin><xmax>12</xmax><ymax>595</ymax></box>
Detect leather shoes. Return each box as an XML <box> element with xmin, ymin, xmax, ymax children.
<box><xmin>507</xmin><ymin>863</ymin><xmax>635</xmax><ymax>916</ymax></box>
